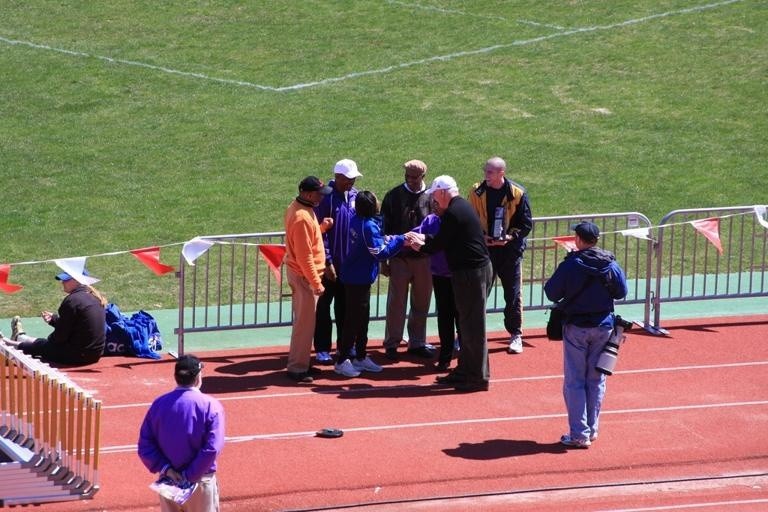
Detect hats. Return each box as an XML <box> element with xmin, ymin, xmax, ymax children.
<box><xmin>176</xmin><ymin>354</ymin><xmax>204</xmax><ymax>377</ymax></box>
<box><xmin>299</xmin><ymin>175</ymin><xmax>333</xmax><ymax>195</ymax></box>
<box><xmin>426</xmin><ymin>174</ymin><xmax>456</xmax><ymax>195</ymax></box>
<box><xmin>402</xmin><ymin>159</ymin><xmax>427</xmax><ymax>174</ymax></box>
<box><xmin>56</xmin><ymin>270</ymin><xmax>88</xmax><ymax>281</ymax></box>
<box><xmin>333</xmin><ymin>159</ymin><xmax>363</xmax><ymax>180</ymax></box>
<box><xmin>571</xmin><ymin>219</ymin><xmax>600</xmax><ymax>241</ymax></box>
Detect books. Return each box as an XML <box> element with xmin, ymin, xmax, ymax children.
<box><xmin>148</xmin><ymin>474</ymin><xmax>199</xmax><ymax>506</ymax></box>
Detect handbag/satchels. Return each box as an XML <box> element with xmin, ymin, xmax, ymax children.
<box><xmin>547</xmin><ymin>301</ymin><xmax>564</xmax><ymax>341</ymax></box>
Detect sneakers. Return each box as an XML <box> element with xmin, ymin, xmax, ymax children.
<box><xmin>317</xmin><ymin>347</ymin><xmax>383</xmax><ymax>377</ymax></box>
<box><xmin>508</xmin><ymin>334</ymin><xmax>523</xmax><ymax>353</ymax></box>
<box><xmin>438</xmin><ymin>375</ymin><xmax>489</xmax><ymax>392</ymax></box>
<box><xmin>409</xmin><ymin>346</ymin><xmax>435</xmax><ymax>357</ymax></box>
<box><xmin>289</xmin><ymin>365</ymin><xmax>320</xmax><ymax>383</ymax></box>
<box><xmin>436</xmin><ymin>362</ymin><xmax>449</xmax><ymax>371</ymax></box>
<box><xmin>11</xmin><ymin>316</ymin><xmax>27</xmax><ymax>341</ymax></box>
<box><xmin>386</xmin><ymin>349</ymin><xmax>398</xmax><ymax>362</ymax></box>
<box><xmin>562</xmin><ymin>433</ymin><xmax>591</xmax><ymax>448</ymax></box>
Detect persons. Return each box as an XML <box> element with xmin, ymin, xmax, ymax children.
<box><xmin>465</xmin><ymin>157</ymin><xmax>532</xmax><ymax>355</ymax></box>
<box><xmin>282</xmin><ymin>174</ymin><xmax>334</xmax><ymax>383</ymax></box>
<box><xmin>542</xmin><ymin>218</ymin><xmax>629</xmax><ymax>448</ymax></box>
<box><xmin>401</xmin><ymin>191</ymin><xmax>461</xmax><ymax>373</ymax></box>
<box><xmin>0</xmin><ymin>266</ymin><xmax>108</xmax><ymax>368</ymax></box>
<box><xmin>313</xmin><ymin>158</ymin><xmax>365</xmax><ymax>364</ymax></box>
<box><xmin>329</xmin><ymin>190</ymin><xmax>414</xmax><ymax>379</ymax></box>
<box><xmin>135</xmin><ymin>352</ymin><xmax>227</xmax><ymax>511</ymax></box>
<box><xmin>379</xmin><ymin>158</ymin><xmax>434</xmax><ymax>362</ymax></box>
<box><xmin>405</xmin><ymin>174</ymin><xmax>495</xmax><ymax>394</ymax></box>
